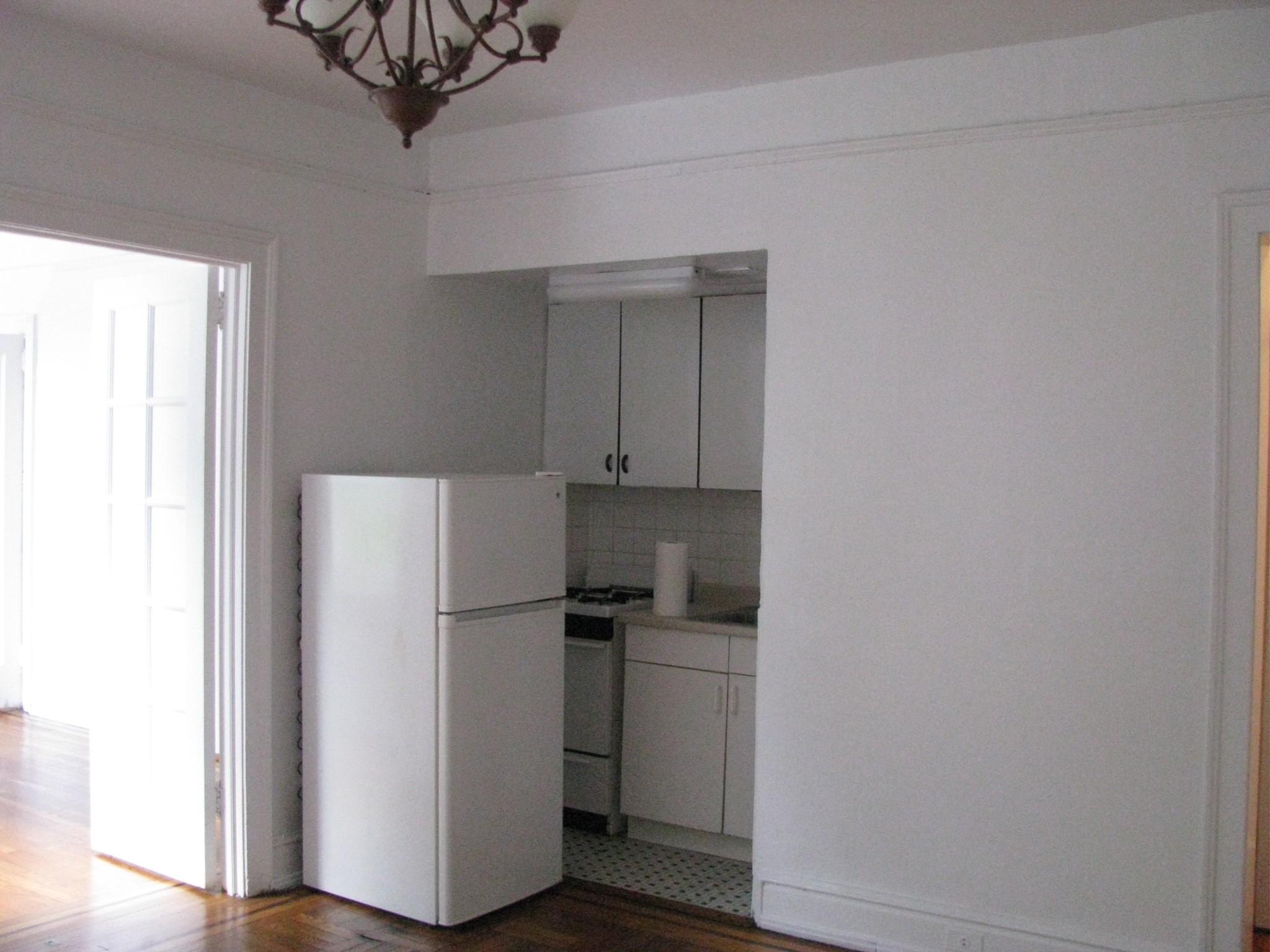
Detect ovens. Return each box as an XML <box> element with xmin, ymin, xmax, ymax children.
<box><xmin>564</xmin><ymin>614</ymin><xmax>629</xmax><ymax>836</ymax></box>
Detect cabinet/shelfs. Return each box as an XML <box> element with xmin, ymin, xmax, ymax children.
<box><xmin>545</xmin><ymin>296</ymin><xmax>700</xmax><ymax>489</ymax></box>
<box><xmin>701</xmin><ymin>294</ymin><xmax>766</xmax><ymax>489</ymax></box>
<box><xmin>622</xmin><ymin>624</ymin><xmax>758</xmax><ymax>862</ymax></box>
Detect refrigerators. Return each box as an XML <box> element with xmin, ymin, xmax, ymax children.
<box><xmin>301</xmin><ymin>470</ymin><xmax>563</xmax><ymax>927</ymax></box>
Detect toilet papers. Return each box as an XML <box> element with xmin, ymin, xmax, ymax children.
<box><xmin>652</xmin><ymin>540</ymin><xmax>689</xmax><ymax>617</ymax></box>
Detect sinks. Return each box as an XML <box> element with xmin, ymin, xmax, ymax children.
<box><xmin>686</xmin><ymin>606</ymin><xmax>757</xmax><ymax>628</ymax></box>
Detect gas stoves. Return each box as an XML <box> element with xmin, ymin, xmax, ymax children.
<box><xmin>566</xmin><ymin>584</ymin><xmax>654</xmax><ymax>618</ymax></box>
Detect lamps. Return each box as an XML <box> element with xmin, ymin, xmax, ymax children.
<box><xmin>254</xmin><ymin>0</ymin><xmax>580</xmax><ymax>151</ymax></box>
